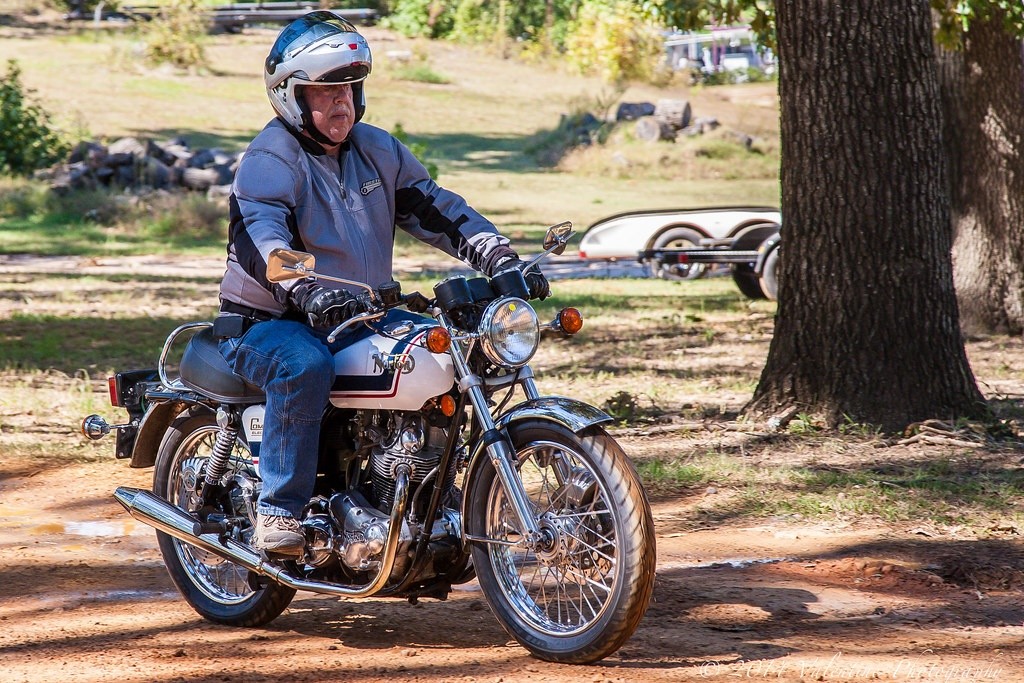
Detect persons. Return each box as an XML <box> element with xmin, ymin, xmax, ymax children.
<box><xmin>213</xmin><ymin>9</ymin><xmax>551</xmax><ymax>555</ymax></box>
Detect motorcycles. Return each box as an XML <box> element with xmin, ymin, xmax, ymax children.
<box><xmin>79</xmin><ymin>222</ymin><xmax>656</xmax><ymax>664</ymax></box>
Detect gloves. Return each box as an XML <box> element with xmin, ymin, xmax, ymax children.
<box><xmin>482</xmin><ymin>245</ymin><xmax>549</xmax><ymax>301</ymax></box>
<box><xmin>291</xmin><ymin>282</ymin><xmax>359</xmax><ymax>329</ymax></box>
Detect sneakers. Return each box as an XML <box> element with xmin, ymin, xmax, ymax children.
<box><xmin>250</xmin><ymin>512</ymin><xmax>305</xmax><ymax>555</ymax></box>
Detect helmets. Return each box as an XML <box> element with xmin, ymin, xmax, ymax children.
<box><xmin>264</xmin><ymin>20</ymin><xmax>372</xmax><ymax>132</ymax></box>
